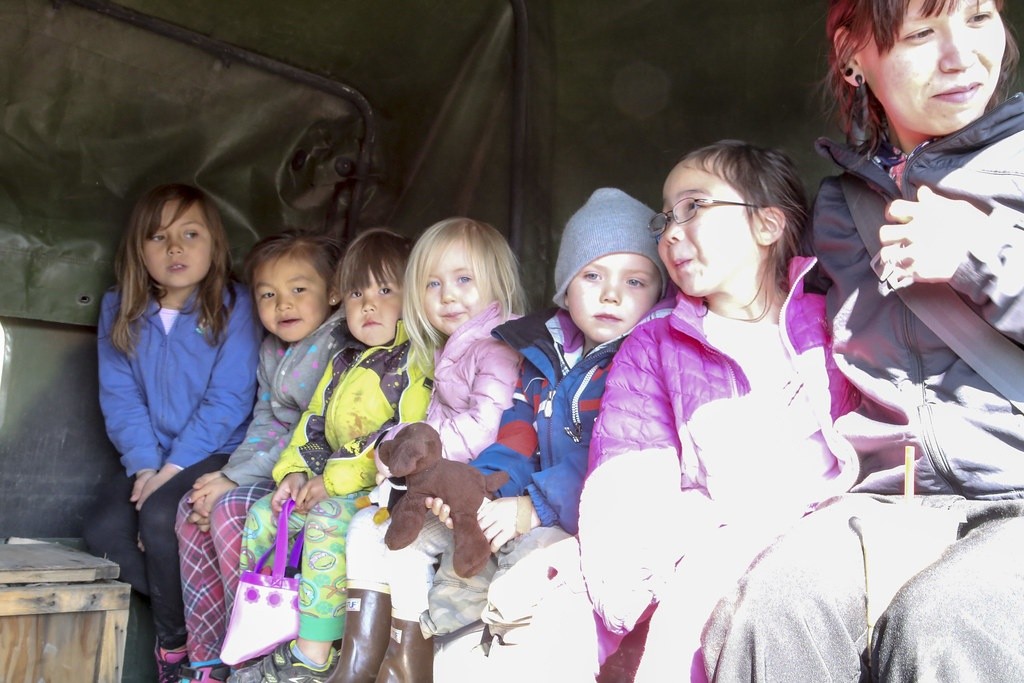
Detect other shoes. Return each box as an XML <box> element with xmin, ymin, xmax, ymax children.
<box><xmin>176</xmin><ymin>658</ymin><xmax>230</xmax><ymax>683</ymax></box>
<box><xmin>154</xmin><ymin>635</ymin><xmax>187</xmax><ymax>683</ymax></box>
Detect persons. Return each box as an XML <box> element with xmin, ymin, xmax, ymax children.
<box><xmin>226</xmin><ymin>227</ymin><xmax>446</xmax><ymax>683</ymax></box>
<box><xmin>424</xmin><ymin>186</ymin><xmax>670</xmax><ymax>683</ymax></box>
<box><xmin>577</xmin><ymin>140</ymin><xmax>860</xmax><ymax>683</ymax></box>
<box><xmin>174</xmin><ymin>229</ymin><xmax>346</xmax><ymax>683</ymax></box>
<box><xmin>701</xmin><ymin>0</ymin><xmax>1024</xmax><ymax>682</ymax></box>
<box><xmin>332</xmin><ymin>216</ymin><xmax>531</xmax><ymax>683</ymax></box>
<box><xmin>83</xmin><ymin>183</ymin><xmax>265</xmax><ymax>682</ymax></box>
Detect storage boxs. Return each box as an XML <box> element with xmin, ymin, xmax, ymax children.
<box><xmin>0</xmin><ymin>541</ymin><xmax>132</xmax><ymax>683</ymax></box>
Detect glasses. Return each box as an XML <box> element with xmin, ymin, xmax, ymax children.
<box><xmin>647</xmin><ymin>197</ymin><xmax>766</xmax><ymax>237</ymax></box>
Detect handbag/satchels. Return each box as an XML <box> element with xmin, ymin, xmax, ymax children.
<box><xmin>220</xmin><ymin>497</ymin><xmax>304</xmax><ymax>665</ymax></box>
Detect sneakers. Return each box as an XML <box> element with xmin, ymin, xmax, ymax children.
<box><xmin>226</xmin><ymin>638</ymin><xmax>342</xmax><ymax>683</ymax></box>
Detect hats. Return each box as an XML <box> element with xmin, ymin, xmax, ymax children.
<box><xmin>552</xmin><ymin>187</ymin><xmax>669</xmax><ymax>311</ymax></box>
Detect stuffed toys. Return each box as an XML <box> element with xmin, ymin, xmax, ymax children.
<box><xmin>379</xmin><ymin>422</ymin><xmax>509</xmax><ymax>578</ymax></box>
